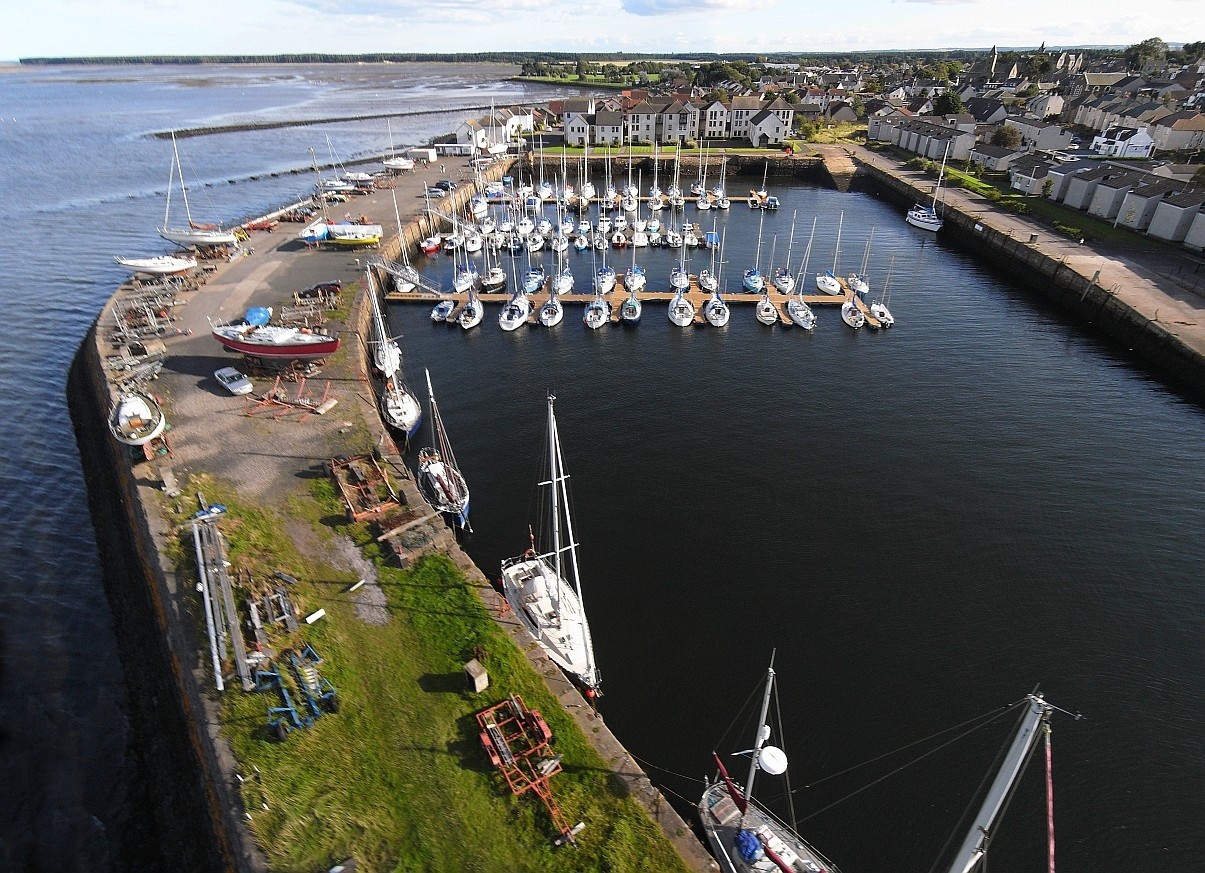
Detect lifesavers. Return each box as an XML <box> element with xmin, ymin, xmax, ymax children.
<box><xmin>525</xmin><ymin>549</ymin><xmax>536</xmax><ymax>558</ymax></box>
<box><xmin>386</xmin><ymin>382</ymin><xmax>394</xmax><ymax>390</ymax></box>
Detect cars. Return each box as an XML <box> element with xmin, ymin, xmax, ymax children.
<box><xmin>423</xmin><ymin>187</ymin><xmax>444</xmax><ymax>199</ymax></box>
<box><xmin>301</xmin><ymin>280</ymin><xmax>343</xmax><ymax>299</ymax></box>
<box><xmin>215</xmin><ymin>364</ymin><xmax>252</xmax><ymax>397</ymax></box>
<box><xmin>436</xmin><ymin>179</ymin><xmax>457</xmax><ymax>191</ymax></box>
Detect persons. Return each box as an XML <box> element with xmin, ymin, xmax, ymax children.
<box><xmin>1078</xmin><ymin>238</ymin><xmax>1084</xmax><ymax>246</ymax></box>
<box><xmin>440</xmin><ymin>164</ymin><xmax>445</xmax><ymax>174</ymax></box>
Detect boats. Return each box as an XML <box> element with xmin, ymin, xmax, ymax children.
<box><xmin>110</xmin><ymin>254</ymin><xmax>195</xmax><ymax>273</ymax></box>
<box><xmin>109</xmin><ymin>386</ymin><xmax>164</xmax><ymax>445</ymax></box>
<box><xmin>210</xmin><ymin>325</ymin><xmax>339</xmax><ymax>358</ymax></box>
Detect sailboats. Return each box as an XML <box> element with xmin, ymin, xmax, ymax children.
<box><xmin>156</xmin><ymin>126</ymin><xmax>237</xmax><ymax>245</ymax></box>
<box><xmin>361</xmin><ymin>94</ymin><xmax>896</xmax><ymax>436</ymax></box>
<box><xmin>697</xmin><ymin>668</ymin><xmax>1062</xmax><ymax>873</ymax></box>
<box><xmin>492</xmin><ymin>394</ymin><xmax>606</xmax><ymax>686</ymax></box>
<box><xmin>416</xmin><ymin>365</ymin><xmax>472</xmax><ymax>529</ymax></box>
<box><xmin>904</xmin><ymin>137</ymin><xmax>950</xmax><ymax>234</ymax></box>
<box><xmin>383</xmin><ymin>119</ymin><xmax>413</xmax><ymax>171</ymax></box>
<box><xmin>294</xmin><ymin>134</ymin><xmax>378</xmax><ymax>248</ymax></box>
